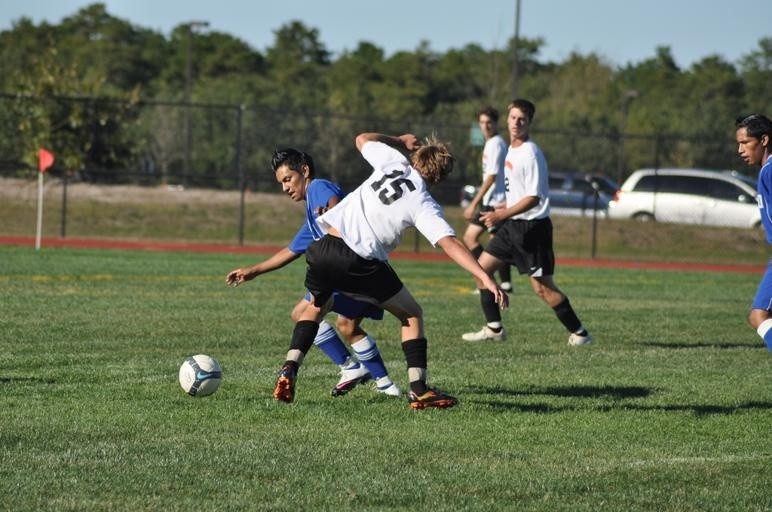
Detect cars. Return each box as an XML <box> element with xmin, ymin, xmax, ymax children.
<box><xmin>458</xmin><ymin>169</ymin><xmax>620</xmax><ymax>217</ymax></box>
<box><xmin>597</xmin><ymin>164</ymin><xmax>763</xmax><ymax>229</ymax></box>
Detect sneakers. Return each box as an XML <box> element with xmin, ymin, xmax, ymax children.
<box><xmin>407</xmin><ymin>387</ymin><xmax>462</xmax><ymax>410</ymax></box>
<box><xmin>472</xmin><ymin>288</ymin><xmax>481</xmax><ymax>295</ymax></box>
<box><xmin>376</xmin><ymin>381</ymin><xmax>403</xmax><ymax>398</ymax></box>
<box><xmin>273</xmin><ymin>365</ymin><xmax>298</xmax><ymax>404</ymax></box>
<box><xmin>568</xmin><ymin>330</ymin><xmax>593</xmax><ymax>348</ymax></box>
<box><xmin>329</xmin><ymin>361</ymin><xmax>372</xmax><ymax>398</ymax></box>
<box><xmin>460</xmin><ymin>324</ymin><xmax>506</xmax><ymax>342</ymax></box>
<box><xmin>500</xmin><ymin>285</ymin><xmax>512</xmax><ymax>294</ymax></box>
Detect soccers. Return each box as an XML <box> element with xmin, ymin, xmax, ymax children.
<box><xmin>179</xmin><ymin>355</ymin><xmax>221</xmax><ymax>398</ymax></box>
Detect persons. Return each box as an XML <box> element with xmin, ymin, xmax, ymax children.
<box><xmin>275</xmin><ymin>131</ymin><xmax>512</xmax><ymax>409</ymax></box>
<box><xmin>734</xmin><ymin>110</ymin><xmax>771</xmax><ymax>348</ymax></box>
<box><xmin>225</xmin><ymin>149</ymin><xmax>400</xmax><ymax>397</ymax></box>
<box><xmin>457</xmin><ymin>109</ymin><xmax>514</xmax><ymax>296</ymax></box>
<box><xmin>460</xmin><ymin>98</ymin><xmax>597</xmax><ymax>347</ymax></box>
